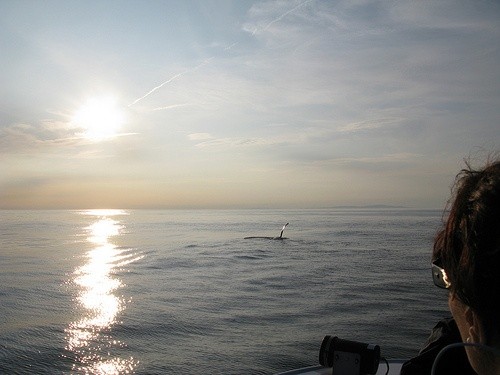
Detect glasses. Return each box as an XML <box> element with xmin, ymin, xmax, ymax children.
<box><xmin>432</xmin><ymin>260</ymin><xmax>456</xmax><ymax>290</ymax></box>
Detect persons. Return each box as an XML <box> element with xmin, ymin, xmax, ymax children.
<box><xmin>431</xmin><ymin>161</ymin><xmax>500</xmax><ymax>375</ymax></box>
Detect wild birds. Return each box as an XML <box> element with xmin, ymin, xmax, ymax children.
<box><xmin>243</xmin><ymin>222</ymin><xmax>291</xmax><ymax>240</ymax></box>
